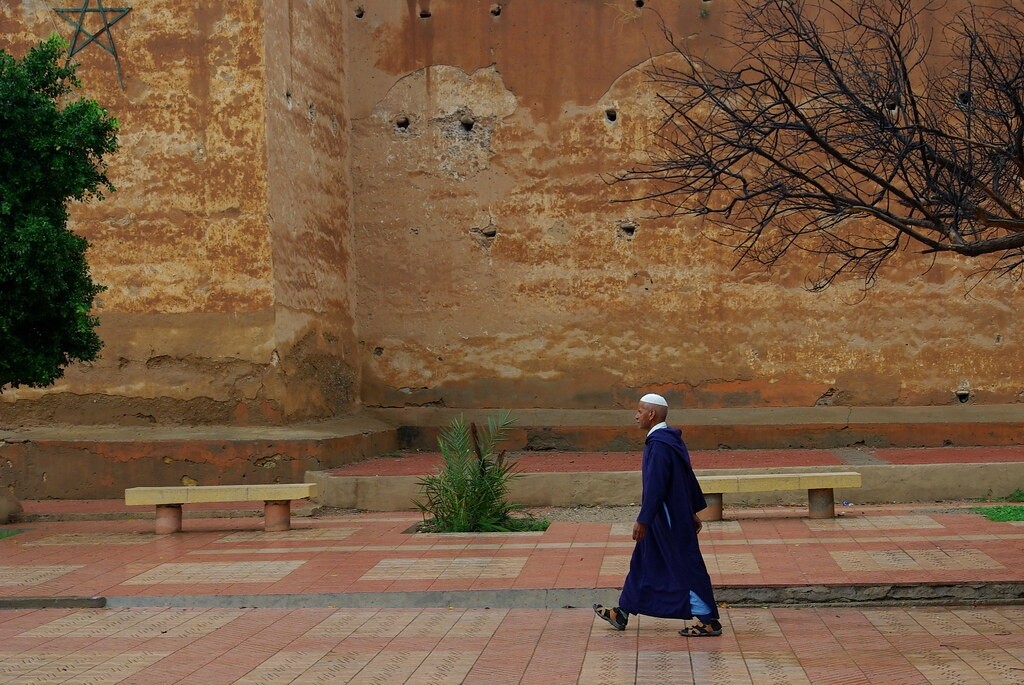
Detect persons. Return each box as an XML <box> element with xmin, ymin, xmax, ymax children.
<box><xmin>593</xmin><ymin>394</ymin><xmax>722</xmax><ymax>637</ymax></box>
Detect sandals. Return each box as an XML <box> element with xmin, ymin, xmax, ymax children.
<box><xmin>678</xmin><ymin>620</ymin><xmax>722</xmax><ymax>636</ymax></box>
<box><xmin>592</xmin><ymin>603</ymin><xmax>628</xmax><ymax>631</ymax></box>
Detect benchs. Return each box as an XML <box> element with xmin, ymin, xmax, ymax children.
<box><xmin>695</xmin><ymin>472</ymin><xmax>862</xmax><ymax>522</ymax></box>
<box><xmin>125</xmin><ymin>483</ymin><xmax>317</xmax><ymax>534</ymax></box>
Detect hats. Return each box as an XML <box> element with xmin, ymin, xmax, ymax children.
<box><xmin>640</xmin><ymin>393</ymin><xmax>668</xmax><ymax>407</ymax></box>
